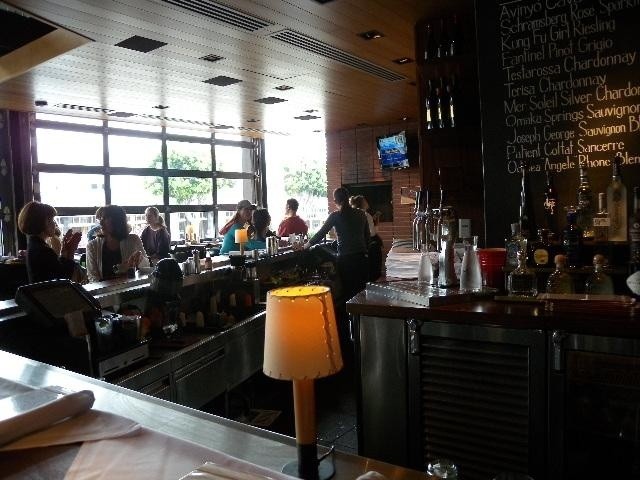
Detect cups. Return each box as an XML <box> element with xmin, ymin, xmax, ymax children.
<box><xmin>266</xmin><ymin>236</ymin><xmax>279</xmax><ymax>255</ymax></box>
<box><xmin>128</xmin><ymin>266</ymin><xmax>136</xmax><ymax>278</ymax></box>
<box><xmin>289</xmin><ymin>231</ymin><xmax>305</xmax><ymax>246</ymax></box>
<box><xmin>197</xmin><ymin>290</ymin><xmax>238</xmax><ymax>326</ymax></box>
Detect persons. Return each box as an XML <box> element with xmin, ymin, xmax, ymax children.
<box><xmin>139</xmin><ymin>206</ymin><xmax>170</xmax><ymax>261</ymax></box>
<box><xmin>219</xmin><ymin>199</ymin><xmax>268</xmax><ymax>258</ymax></box>
<box><xmin>250</xmin><ymin>207</ymin><xmax>271</xmax><ymax>240</ymax></box>
<box><xmin>291</xmin><ymin>186</ymin><xmax>371</xmax><ymax>343</ymax></box>
<box><xmin>276</xmin><ymin>197</ymin><xmax>309</xmax><ymax>237</ymax></box>
<box><xmin>86</xmin><ymin>206</ymin><xmax>105</xmax><ymax>238</ymax></box>
<box><xmin>84</xmin><ymin>203</ymin><xmax>151</xmax><ymax>282</ymax></box>
<box><xmin>17</xmin><ymin>200</ymin><xmax>87</xmax><ymax>284</ymax></box>
<box><xmin>348</xmin><ymin>194</ymin><xmax>383</xmax><ymax>286</ymax></box>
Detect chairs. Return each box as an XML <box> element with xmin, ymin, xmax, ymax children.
<box><xmin>170</xmin><ymin>238</ymin><xmax>215</xmax><ymax>262</ymax></box>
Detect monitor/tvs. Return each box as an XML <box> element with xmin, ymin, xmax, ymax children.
<box><xmin>15</xmin><ymin>278</ymin><xmax>101</xmax><ymax>329</ymax></box>
<box><xmin>376</xmin><ymin>130</ymin><xmax>411</xmax><ymax>171</ymax></box>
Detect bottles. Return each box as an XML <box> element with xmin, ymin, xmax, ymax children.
<box><xmin>561</xmin><ymin>225</ymin><xmax>583</xmax><ymax>263</ymax></box>
<box><xmin>543</xmin><ymin>167</ymin><xmax>560</xmax><ymax>230</ymax></box>
<box><xmin>566</xmin><ymin>205</ymin><xmax>576</xmax><ymax>224</ymax></box>
<box><xmin>606</xmin><ymin>155</ymin><xmax>629</xmax><ymax>241</ymax></box>
<box><xmin>506</xmin><ymin>251</ymin><xmax>539</xmax><ymax>296</ymax></box>
<box><xmin>513</xmin><ymin>156</ymin><xmax>533</xmax><ymax>231</ymax></box>
<box><xmin>583</xmin><ymin>255</ymin><xmax>615</xmax><ymax>298</ymax></box>
<box><xmin>185</xmin><ymin>234</ymin><xmax>188</xmax><ymax>245</ymax></box>
<box><xmin>422</xmin><ymin>13</ymin><xmax>462</xmax><ymax>130</ymax></box>
<box><xmin>205</xmin><ymin>248</ymin><xmax>212</xmax><ymax>270</ymax></box>
<box><xmin>188</xmin><ymin>258</ymin><xmax>195</xmax><ymax>273</ymax></box>
<box><xmin>408</xmin><ymin>187</ymin><xmax>454</xmax><ymax>246</ymax></box>
<box><xmin>191</xmin><ymin>248</ymin><xmax>202</xmax><ymax>274</ymax></box>
<box><xmin>545</xmin><ymin>254</ymin><xmax>574</xmax><ymax>297</ymax></box>
<box><xmin>596</xmin><ymin>192</ymin><xmax>608</xmax><ymax>241</ymax></box>
<box><xmin>503</xmin><ymin>224</ymin><xmax>528</xmax><ymax>261</ymax></box>
<box><xmin>627</xmin><ymin>187</ymin><xmax>640</xmax><ymax>272</ymax></box>
<box><xmin>416</xmin><ymin>235</ymin><xmax>483</xmax><ymax>294</ymax></box>
<box><xmin>572</xmin><ymin>163</ymin><xmax>598</xmax><ymax>234</ymax></box>
<box><xmin>532</xmin><ymin>228</ymin><xmax>553</xmax><ymax>266</ymax></box>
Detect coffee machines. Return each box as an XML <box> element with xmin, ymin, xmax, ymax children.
<box><xmin>242</xmin><ymin>262</ymin><xmax>261</xmax><ymax>310</ymax></box>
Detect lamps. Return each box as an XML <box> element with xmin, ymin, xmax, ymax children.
<box><xmin>234</xmin><ymin>229</ymin><xmax>249</xmax><ymax>254</ymax></box>
<box><xmin>261</xmin><ymin>286</ymin><xmax>344</xmax><ymax>480</ymax></box>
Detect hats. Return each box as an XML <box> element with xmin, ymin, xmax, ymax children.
<box><xmin>237</xmin><ymin>200</ymin><xmax>257</xmax><ymax>210</ymax></box>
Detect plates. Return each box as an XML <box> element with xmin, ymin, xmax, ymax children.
<box><xmin>140</xmin><ymin>266</ymin><xmax>155</xmax><ymax>272</ymax></box>
